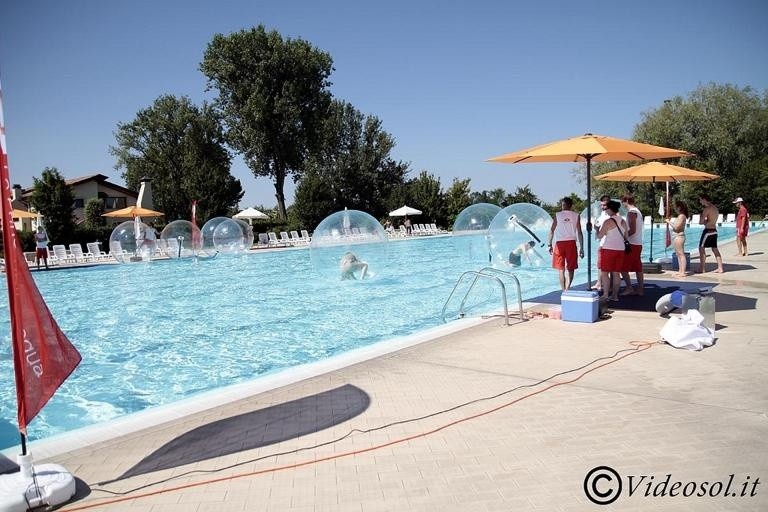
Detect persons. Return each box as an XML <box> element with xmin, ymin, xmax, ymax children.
<box><xmin>337</xmin><ymin>251</ymin><xmax>369</xmax><ymax>282</ymax></box>
<box><xmin>619</xmin><ymin>192</ymin><xmax>645</xmax><ymax>297</ymax></box>
<box><xmin>694</xmin><ymin>192</ymin><xmax>725</xmax><ymax>274</ymax></box>
<box><xmin>32</xmin><ymin>226</ymin><xmax>52</xmax><ymax>271</ymax></box>
<box><xmin>546</xmin><ymin>196</ymin><xmax>585</xmax><ymax>292</ymax></box>
<box><xmin>504</xmin><ymin>239</ymin><xmax>548</xmax><ymax>268</ymax></box>
<box><xmin>135</xmin><ymin>223</ymin><xmax>161</xmax><ymax>258</ymax></box>
<box><xmin>731</xmin><ymin>197</ymin><xmax>750</xmax><ymax>258</ymax></box>
<box><xmin>591</xmin><ymin>194</ymin><xmax>619</xmax><ymax>295</ymax></box>
<box><xmin>384</xmin><ymin>219</ymin><xmax>394</xmax><ymax>235</ymax></box>
<box><xmin>664</xmin><ymin>200</ymin><xmax>690</xmax><ymax>278</ymax></box>
<box><xmin>403</xmin><ymin>218</ymin><xmax>412</xmax><ymax>235</ymax></box>
<box><xmin>594</xmin><ymin>200</ymin><xmax>630</xmax><ymax>302</ymax></box>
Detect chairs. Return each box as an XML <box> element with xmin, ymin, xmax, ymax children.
<box><xmin>35</xmin><ymin>238</ymin><xmax>184</xmax><ymax>266</ymax></box>
<box><xmin>257</xmin><ymin>223</ymin><xmax>442</xmax><ymax>248</ymax></box>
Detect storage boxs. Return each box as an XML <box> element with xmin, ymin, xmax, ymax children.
<box><xmin>560</xmin><ymin>290</ymin><xmax>599</xmax><ymax>322</ymax></box>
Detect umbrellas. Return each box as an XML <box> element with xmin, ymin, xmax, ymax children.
<box><xmin>101</xmin><ymin>204</ymin><xmax>165</xmax><ymax>221</ymax></box>
<box><xmin>657</xmin><ymin>196</ymin><xmax>666</xmax><ymax>223</ymax></box>
<box><xmin>484</xmin><ymin>133</ymin><xmax>697</xmax><ymax>291</ymax></box>
<box><xmin>0</xmin><ymin>206</ymin><xmax>44</xmax><ymax>221</ymax></box>
<box><xmin>388</xmin><ymin>204</ymin><xmax>422</xmax><ymax>221</ymax></box>
<box><xmin>344</xmin><ymin>205</ymin><xmax>351</xmax><ymax>229</ymax></box>
<box><xmin>231</xmin><ymin>206</ymin><xmax>270</xmax><ymax>226</ymax></box>
<box><xmin>592</xmin><ymin>159</ymin><xmax>722</xmax><ymax>265</ymax></box>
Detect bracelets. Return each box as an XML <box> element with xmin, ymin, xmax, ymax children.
<box><xmin>548</xmin><ymin>244</ymin><xmax>551</xmax><ymax>247</ymax></box>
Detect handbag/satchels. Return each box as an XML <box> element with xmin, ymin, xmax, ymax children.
<box><xmin>624</xmin><ymin>240</ymin><xmax>631</xmax><ymax>253</ymax></box>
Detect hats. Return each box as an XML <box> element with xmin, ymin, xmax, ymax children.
<box><xmin>732</xmin><ymin>197</ymin><xmax>743</xmax><ymax>203</ymax></box>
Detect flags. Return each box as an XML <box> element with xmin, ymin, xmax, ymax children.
<box><xmin>0</xmin><ymin>90</ymin><xmax>81</xmax><ymax>434</ymax></box>
<box><xmin>191</xmin><ymin>199</ymin><xmax>201</xmax><ymax>250</ymax></box>
